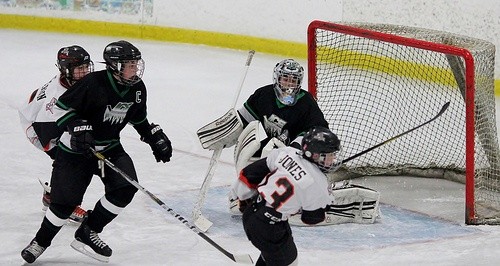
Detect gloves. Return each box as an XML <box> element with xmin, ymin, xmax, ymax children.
<box><xmin>140</xmin><ymin>122</ymin><xmax>173</xmax><ymax>163</ymax></box>
<box><xmin>69</xmin><ymin>119</ymin><xmax>96</xmax><ymax>154</ymax></box>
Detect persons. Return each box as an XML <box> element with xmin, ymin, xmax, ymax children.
<box><xmin>19</xmin><ymin>40</ymin><xmax>173</xmax><ymax>265</ymax></box>
<box><xmin>197</xmin><ymin>60</ymin><xmax>379</xmax><ymax>227</ymax></box>
<box><xmin>236</xmin><ymin>127</ymin><xmax>342</xmax><ymax>265</ymax></box>
<box><xmin>19</xmin><ymin>45</ymin><xmax>93</xmax><ymax>227</ymax></box>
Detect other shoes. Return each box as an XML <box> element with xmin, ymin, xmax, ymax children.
<box><xmin>67</xmin><ymin>206</ymin><xmax>87</xmax><ymax>224</ymax></box>
<box><xmin>42</xmin><ymin>182</ymin><xmax>52</xmax><ymax>211</ymax></box>
<box><xmin>229</xmin><ymin>197</ymin><xmax>243</xmax><ymax>220</ymax></box>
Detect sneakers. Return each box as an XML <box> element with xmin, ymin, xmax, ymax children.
<box><xmin>20</xmin><ymin>223</ymin><xmax>55</xmax><ymax>265</ymax></box>
<box><xmin>70</xmin><ymin>217</ymin><xmax>112</xmax><ymax>264</ymax></box>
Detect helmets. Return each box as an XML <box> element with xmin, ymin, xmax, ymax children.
<box><xmin>272</xmin><ymin>59</ymin><xmax>304</xmax><ymax>106</ymax></box>
<box><xmin>301</xmin><ymin>126</ymin><xmax>340</xmax><ymax>173</ymax></box>
<box><xmin>54</xmin><ymin>45</ymin><xmax>94</xmax><ymax>87</ymax></box>
<box><xmin>103</xmin><ymin>40</ymin><xmax>145</xmax><ymax>86</ymax></box>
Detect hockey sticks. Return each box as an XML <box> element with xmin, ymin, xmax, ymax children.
<box><xmin>91</xmin><ymin>146</ymin><xmax>255</xmax><ymax>265</ymax></box>
<box><xmin>334</xmin><ymin>100</ymin><xmax>450</xmax><ymax>166</ymax></box>
<box><xmin>37</xmin><ymin>175</ymin><xmax>52</xmax><ymax>193</ymax></box>
<box><xmin>192</xmin><ymin>50</ymin><xmax>256</xmax><ymax>232</ymax></box>
